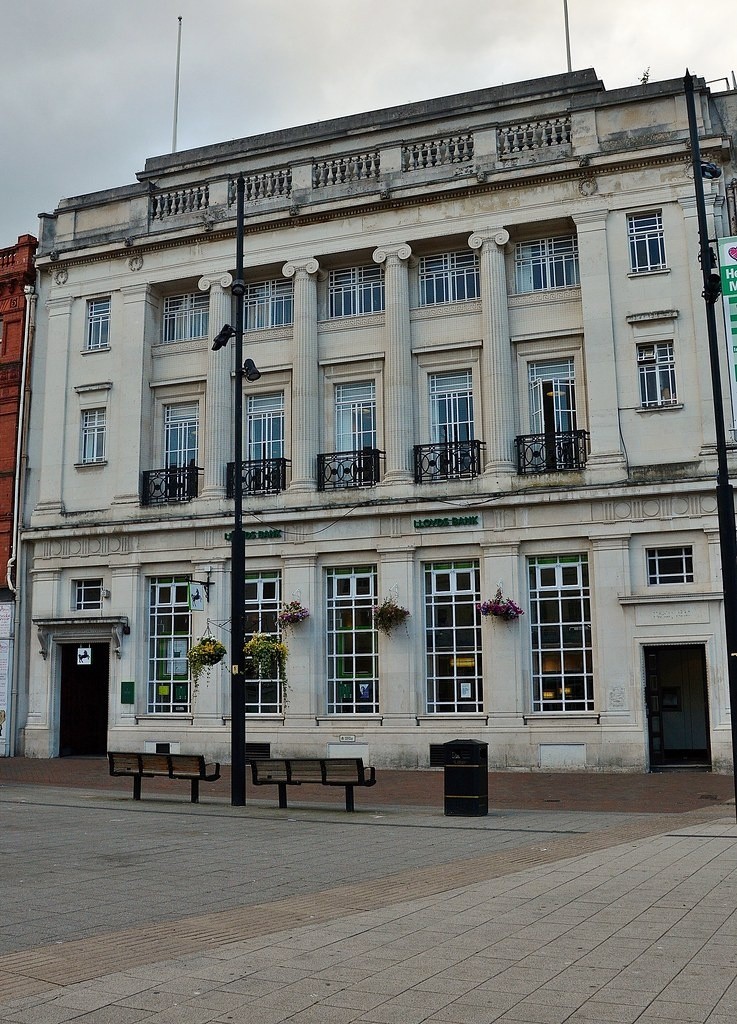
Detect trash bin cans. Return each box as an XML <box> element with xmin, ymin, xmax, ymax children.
<box><xmin>442</xmin><ymin>738</ymin><xmax>490</xmax><ymax>817</ymax></box>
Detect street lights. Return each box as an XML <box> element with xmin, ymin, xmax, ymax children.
<box><xmin>212</xmin><ymin>174</ymin><xmax>262</xmax><ymax>809</ymax></box>
<box><xmin>684</xmin><ymin>69</ymin><xmax>736</xmax><ymax>804</ymax></box>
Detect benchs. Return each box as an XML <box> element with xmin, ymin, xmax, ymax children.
<box><xmin>107</xmin><ymin>751</ymin><xmax>221</xmax><ymax>804</ymax></box>
<box><xmin>250</xmin><ymin>758</ymin><xmax>376</xmax><ymax>813</ymax></box>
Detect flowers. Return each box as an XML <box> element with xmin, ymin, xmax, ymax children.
<box><xmin>187</xmin><ymin>636</ymin><xmax>227</xmax><ymax>701</ymax></box>
<box><xmin>476</xmin><ymin>589</ymin><xmax>524</xmax><ymax>633</ymax></box>
<box><xmin>276</xmin><ymin>599</ymin><xmax>309</xmax><ymax>640</ymax></box>
<box><xmin>370</xmin><ymin>594</ymin><xmax>413</xmax><ymax>641</ymax></box>
<box><xmin>243</xmin><ymin>633</ymin><xmax>293</xmax><ymax>717</ymax></box>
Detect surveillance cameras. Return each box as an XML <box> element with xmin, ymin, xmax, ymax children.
<box><xmin>212</xmin><ymin>324</ymin><xmax>233</xmax><ymax>351</ymax></box>
<box><xmin>702</xmin><ymin>165</ymin><xmax>721</xmax><ymax>179</ymax></box>
